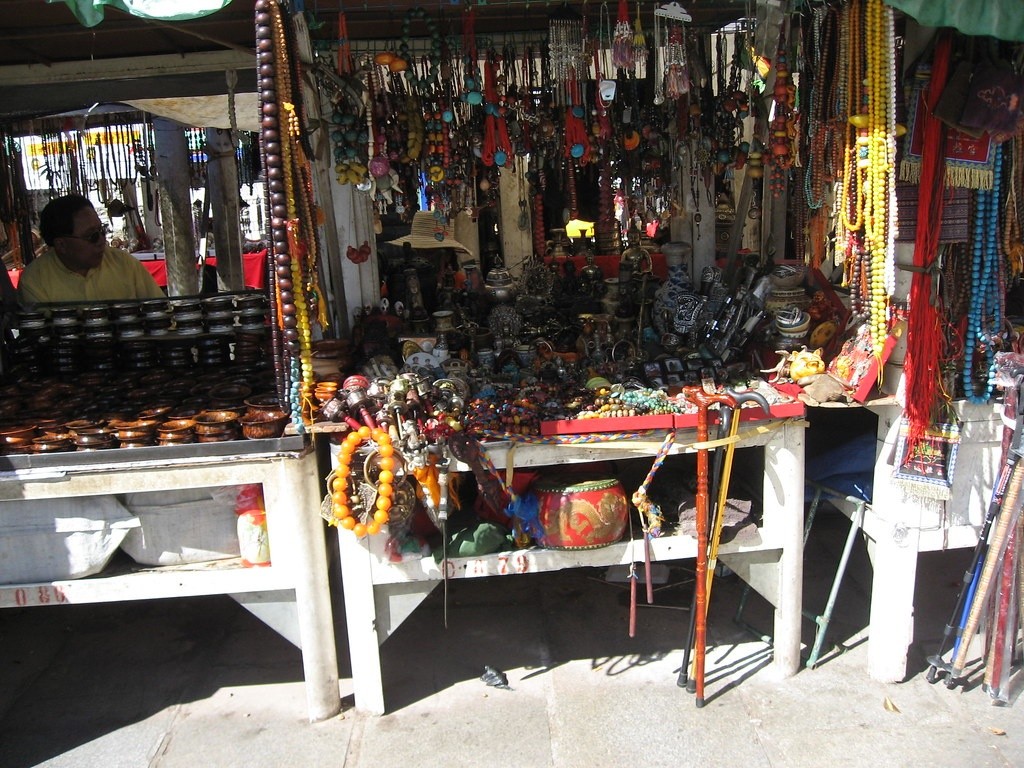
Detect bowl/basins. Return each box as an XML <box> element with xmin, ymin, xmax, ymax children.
<box><xmin>464</xmin><ymin>327</ymin><xmax>493</xmax><ymax>349</ymax></box>
<box><xmin>776</xmin><ymin>312</ymin><xmax>812</xmax><ymax>339</ymax></box>
<box><xmin>0</xmin><ymin>363</ymin><xmax>291</xmax><ymax>454</ymax></box>
<box><xmin>311</xmin><ymin>339</ymin><xmax>352</xmax><ymax>358</ymax></box>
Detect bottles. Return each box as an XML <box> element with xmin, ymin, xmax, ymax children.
<box><xmin>431</xmin><ymin>310</ymin><xmax>459</xmax><ymax>336</ymax></box>
<box><xmin>654</xmin><ymin>241</ymin><xmax>699</xmax><ymax>339</ymax></box>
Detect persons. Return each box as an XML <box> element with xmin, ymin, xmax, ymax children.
<box><xmin>18</xmin><ymin>194</ymin><xmax>166</xmax><ymax>303</ymax></box>
<box><xmin>404</xmin><ymin>276</ymin><xmax>428</xmax><ymax>320</ymax></box>
<box><xmin>580</xmin><ymin>249</ymin><xmax>602</xmax><ymax>282</ymax></box>
<box><xmin>621</xmin><ymin>228</ymin><xmax>652</xmax><ymax>274</ymax></box>
<box><xmin>806</xmin><ymin>290</ymin><xmax>832</xmax><ymax>322</ymax></box>
<box><xmin>425</xmin><ymin>359</ymin><xmax>434</xmax><ymax>369</ymax></box>
<box><xmin>412</xmin><ymin>356</ymin><xmax>419</xmax><ymax>364</ymax></box>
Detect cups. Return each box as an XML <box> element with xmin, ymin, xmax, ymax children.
<box><xmin>477</xmin><ymin>348</ymin><xmax>494</xmax><ymax>365</ymax></box>
<box><xmin>315</xmin><ymin>382</ymin><xmax>339</xmax><ymax>402</ymax></box>
<box><xmin>516</xmin><ymin>344</ymin><xmax>537</xmax><ymax>367</ymax></box>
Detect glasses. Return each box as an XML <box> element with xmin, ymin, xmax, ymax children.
<box><xmin>58</xmin><ymin>223</ymin><xmax>108</xmax><ymax>244</ymax></box>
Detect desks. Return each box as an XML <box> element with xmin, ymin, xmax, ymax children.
<box><xmin>0</xmin><ymin>441</ymin><xmax>341</xmax><ymax>722</ymax></box>
<box><xmin>330</xmin><ymin>416</ymin><xmax>810</xmax><ymax>717</ymax></box>
<box><xmin>828</xmin><ymin>399</ymin><xmax>1023</xmax><ymax>685</ymax></box>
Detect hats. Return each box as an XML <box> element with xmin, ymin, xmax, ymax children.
<box><xmin>385</xmin><ymin>208</ymin><xmax>473</xmax><ymax>258</ymax></box>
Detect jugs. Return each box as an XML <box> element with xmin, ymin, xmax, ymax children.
<box><xmin>581</xmin><ymin>333</ymin><xmax>612</xmax><ymax>364</ymax></box>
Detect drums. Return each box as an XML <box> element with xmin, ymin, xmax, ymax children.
<box><xmin>530</xmin><ymin>478</ymin><xmax>628</xmax><ymax>551</ymax></box>
